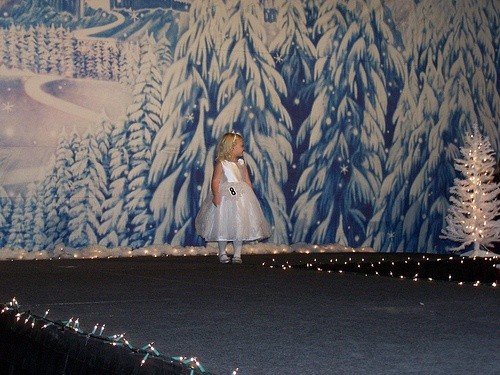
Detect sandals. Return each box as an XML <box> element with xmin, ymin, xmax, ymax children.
<box><xmin>219</xmin><ymin>253</ymin><xmax>231</xmax><ymax>264</ymax></box>
<box><xmin>232</xmin><ymin>256</ymin><xmax>243</xmax><ymax>264</ymax></box>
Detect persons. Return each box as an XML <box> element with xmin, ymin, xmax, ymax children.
<box><xmin>195</xmin><ymin>133</ymin><xmax>272</xmax><ymax>263</ymax></box>
